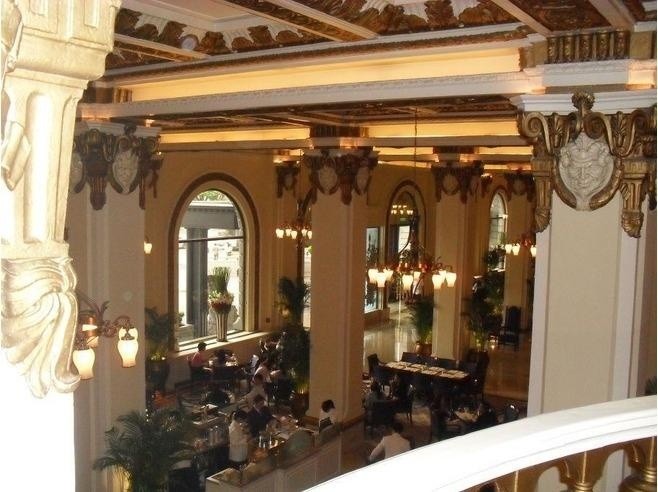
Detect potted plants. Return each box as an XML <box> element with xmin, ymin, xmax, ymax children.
<box><xmin>280</xmin><ymin>326</ymin><xmax>311</xmax><ymax>421</ymax></box>
<box><xmin>402</xmin><ymin>294</ymin><xmax>434</xmax><ymax>352</ymax></box>
<box><xmin>145</xmin><ymin>309</ymin><xmax>186</xmax><ymax>385</ymax></box>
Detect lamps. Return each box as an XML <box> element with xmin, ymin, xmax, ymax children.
<box><xmin>277</xmin><ymin>150</ymin><xmax>313</xmax><ymax>244</ymax></box>
<box><xmin>369</xmin><ymin>106</ymin><xmax>458</xmax><ymax>291</ymax></box>
<box><xmin>73</xmin><ymin>289</ymin><xmax>139</xmax><ymax>382</ymax></box>
<box><xmin>503</xmin><ymin>174</ymin><xmax>539</xmax><ymax>255</ymax></box>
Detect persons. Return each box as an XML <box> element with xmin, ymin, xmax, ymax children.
<box><xmin>236</xmin><ymin>332</ymin><xmax>310</xmax><ymax>440</ymax></box>
<box><xmin>368</xmin><ymin>420</ymin><xmax>411</xmax><ymax>464</ymax></box>
<box><xmin>228</xmin><ymin>408</ymin><xmax>252</xmax><ymax>469</ymax></box>
<box><xmin>202</xmin><ymin>382</ymin><xmax>231</xmax><ymax>410</ymax></box>
<box><xmin>212</xmin><ymin>349</ymin><xmax>232</xmax><ymax>392</ymax></box>
<box><xmin>318</xmin><ymin>399</ymin><xmax>338</xmax><ymax>434</ymax></box>
<box><xmin>432</xmin><ymin>393</ymin><xmax>467</xmax><ymax>436</ymax></box>
<box><xmin>366</xmin><ymin>381</ymin><xmax>388</xmax><ymax>431</ymax></box>
<box><xmin>467</xmin><ymin>399</ymin><xmax>492</xmax><ymax>433</ymax></box>
<box><xmin>193</xmin><ymin>341</ymin><xmax>216</xmax><ymax>388</ymax></box>
<box><xmin>389</xmin><ymin>372</ymin><xmax>410</xmax><ymax>414</ymax></box>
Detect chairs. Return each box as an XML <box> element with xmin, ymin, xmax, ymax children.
<box><xmin>497</xmin><ymin>306</ymin><xmax>522</xmax><ymax>350</ymax></box>
<box><xmin>183</xmin><ymin>346</ymin><xmax>303</xmax><ymax>442</ymax></box>
<box><xmin>362</xmin><ymin>346</ymin><xmax>522</xmax><ymax>441</ymax></box>
